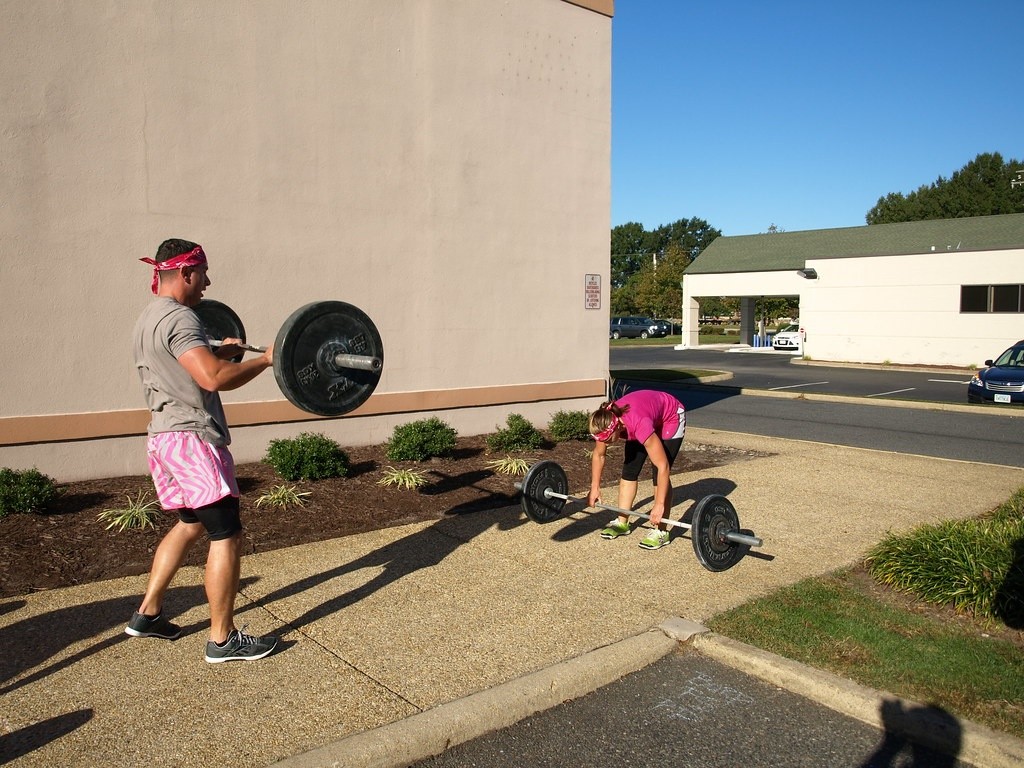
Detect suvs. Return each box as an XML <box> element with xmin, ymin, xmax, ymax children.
<box><xmin>611</xmin><ymin>316</ymin><xmax>668</xmax><ymax>340</ymax></box>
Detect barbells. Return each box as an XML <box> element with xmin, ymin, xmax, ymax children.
<box><xmin>189</xmin><ymin>297</ymin><xmax>385</xmax><ymax>418</ymax></box>
<box><xmin>512</xmin><ymin>458</ymin><xmax>764</xmax><ymax>573</ymax></box>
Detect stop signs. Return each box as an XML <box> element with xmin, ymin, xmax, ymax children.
<box><xmin>800</xmin><ymin>327</ymin><xmax>805</xmax><ymax>333</ymax></box>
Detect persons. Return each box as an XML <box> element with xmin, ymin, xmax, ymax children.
<box><xmin>125</xmin><ymin>238</ymin><xmax>279</xmax><ymax>664</ymax></box>
<box><xmin>586</xmin><ymin>390</ymin><xmax>686</xmax><ymax>549</ymax></box>
<box><xmin>756</xmin><ymin>314</ymin><xmax>774</xmax><ymax>327</ymax></box>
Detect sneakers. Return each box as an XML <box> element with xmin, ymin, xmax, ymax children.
<box><xmin>600</xmin><ymin>518</ymin><xmax>631</xmax><ymax>540</ymax></box>
<box><xmin>203</xmin><ymin>628</ymin><xmax>279</xmax><ymax>664</ymax></box>
<box><xmin>125</xmin><ymin>610</ymin><xmax>183</xmax><ymax>640</ymax></box>
<box><xmin>638</xmin><ymin>528</ymin><xmax>671</xmax><ymax>550</ymax></box>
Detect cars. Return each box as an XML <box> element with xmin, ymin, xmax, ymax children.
<box><xmin>967</xmin><ymin>340</ymin><xmax>1024</xmax><ymax>406</ymax></box>
<box><xmin>773</xmin><ymin>324</ymin><xmax>799</xmax><ymax>350</ymax></box>
<box><xmin>652</xmin><ymin>319</ymin><xmax>674</xmax><ymax>334</ymax></box>
